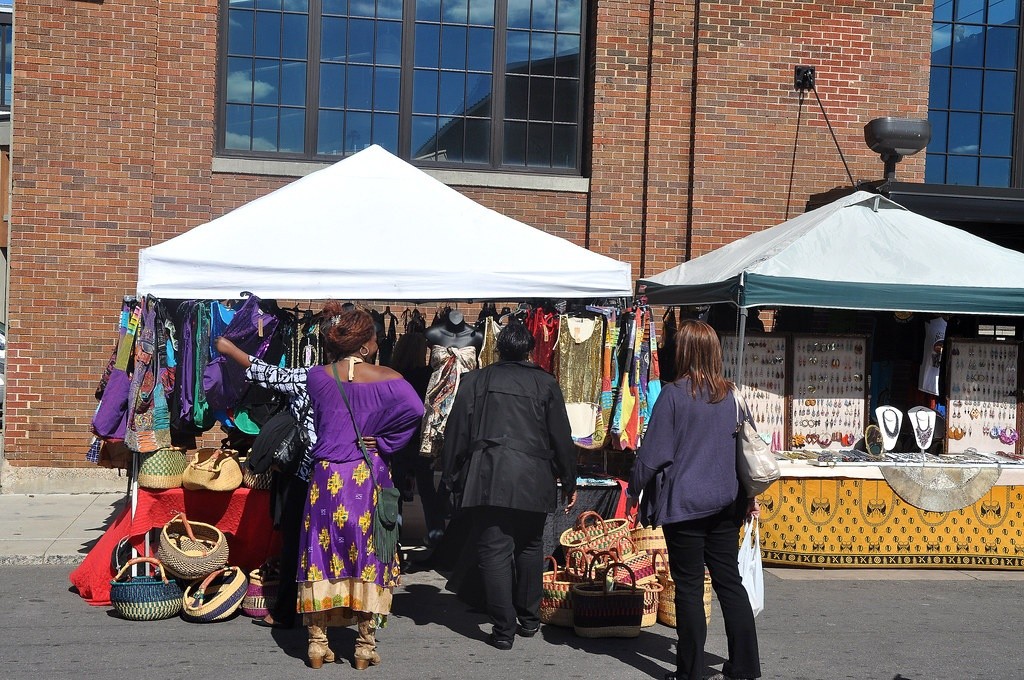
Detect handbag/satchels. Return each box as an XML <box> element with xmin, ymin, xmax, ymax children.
<box><xmin>372</xmin><ymin>488</ymin><xmax>400</xmax><ymax>564</ymax></box>
<box><xmin>732</xmin><ymin>382</ymin><xmax>781</xmax><ymax>499</ymax></box>
<box><xmin>571</xmin><ymin>304</ymin><xmax>661</xmax><ymax>451</ymax></box>
<box><xmin>92</xmin><ymin>293</ymin><xmax>171</xmax><ymax>453</ymax></box>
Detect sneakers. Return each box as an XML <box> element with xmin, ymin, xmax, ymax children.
<box><xmin>519</xmin><ymin>622</ymin><xmax>540</xmax><ymax>637</ymax></box>
<box><xmin>493</xmin><ymin>632</ymin><xmax>514</xmax><ymax>650</ymax></box>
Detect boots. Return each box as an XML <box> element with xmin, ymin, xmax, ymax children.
<box><xmin>307</xmin><ymin>611</ymin><xmax>337</xmax><ymax>669</ymax></box>
<box><xmin>354</xmin><ymin>613</ymin><xmax>382</xmax><ymax>670</ymax></box>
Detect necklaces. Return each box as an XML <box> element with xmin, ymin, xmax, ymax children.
<box><xmin>883</xmin><ymin>408</ymin><xmax>899</xmax><ymax>437</ymax></box>
<box><xmin>781</xmin><ymin>448</ymin><xmax>1024</xmax><ymax>465</ymax></box>
<box><xmin>915</xmin><ymin>408</ymin><xmax>932</xmax><ymax>448</ymax></box>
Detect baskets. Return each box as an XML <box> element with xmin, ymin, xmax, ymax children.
<box><xmin>182</xmin><ymin>447</ymin><xmax>243</xmax><ymax>491</ymax></box>
<box><xmin>243</xmin><ymin>448</ymin><xmax>272</xmax><ymax>490</ymax></box>
<box><xmin>541</xmin><ymin>511</ymin><xmax>712</xmax><ymax>638</ymax></box>
<box><xmin>157</xmin><ymin>512</ymin><xmax>229</xmax><ymax>579</ymax></box>
<box><xmin>109</xmin><ymin>557</ymin><xmax>183</xmax><ymax>621</ymax></box>
<box><xmin>138</xmin><ymin>445</ymin><xmax>186</xmax><ymax>489</ymax></box>
<box><xmin>183</xmin><ymin>566</ymin><xmax>248</xmax><ymax>621</ymax></box>
<box><xmin>242</xmin><ymin>569</ymin><xmax>269</xmax><ymax>616</ymax></box>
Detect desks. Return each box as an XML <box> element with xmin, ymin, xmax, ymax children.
<box><xmin>738</xmin><ymin>452</ymin><xmax>1024</xmax><ymax>571</ymax></box>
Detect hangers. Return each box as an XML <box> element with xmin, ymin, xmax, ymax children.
<box><xmin>211</xmin><ymin>300</ymin><xmax>652</xmax><ymax>343</ymax></box>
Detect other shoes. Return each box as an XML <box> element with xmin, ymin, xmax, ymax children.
<box><xmin>723</xmin><ymin>675</ymin><xmax>756</xmax><ymax>680</ymax></box>
<box><xmin>663</xmin><ymin>672</ymin><xmax>703</xmax><ymax>680</ymax></box>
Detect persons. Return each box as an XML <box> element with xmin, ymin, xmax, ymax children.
<box><xmin>384</xmin><ymin>333</ymin><xmax>445</xmax><ymax>545</ymax></box>
<box><xmin>626</xmin><ymin>319</ymin><xmax>761</xmax><ymax>680</ymax></box>
<box><xmin>295</xmin><ymin>302</ymin><xmax>424</xmax><ymax>670</ymax></box>
<box><xmin>420</xmin><ymin>312</ymin><xmax>484</xmax><ymax>457</ymax></box>
<box><xmin>213</xmin><ymin>335</ymin><xmax>340</xmax><ymax>628</ymax></box>
<box><xmin>440</xmin><ymin>324</ymin><xmax>578</xmax><ymax>650</ymax></box>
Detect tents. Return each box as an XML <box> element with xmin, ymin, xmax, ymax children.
<box><xmin>130</xmin><ymin>143</ymin><xmax>633</xmax><ymax>578</ymax></box>
<box><xmin>634</xmin><ymin>190</ymin><xmax>1024</xmax><ymax>392</ymax></box>
